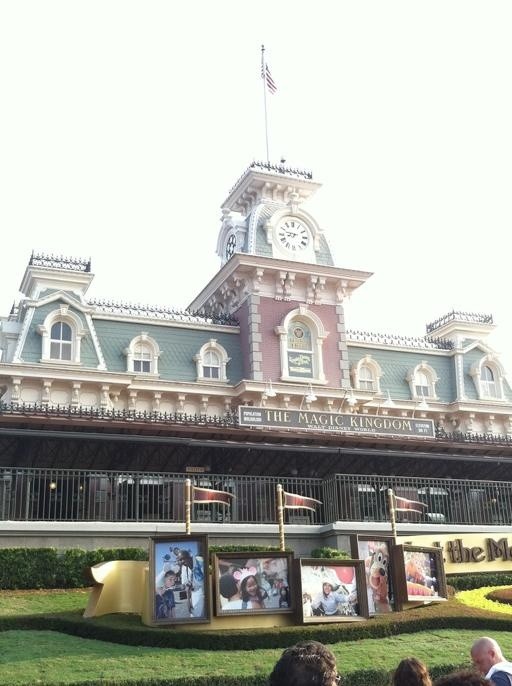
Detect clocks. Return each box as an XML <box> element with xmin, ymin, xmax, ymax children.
<box><xmin>274</xmin><ymin>215</ymin><xmax>314</xmax><ymax>258</ymax></box>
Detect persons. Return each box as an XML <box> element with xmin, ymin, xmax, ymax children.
<box><xmin>394</xmin><ymin>658</ymin><xmax>432</xmax><ymax>686</ymax></box>
<box><xmin>470</xmin><ymin>637</ymin><xmax>512</xmax><ymax>686</ymax></box>
<box><xmin>156</xmin><ymin>546</ymin><xmax>205</xmax><ymax>620</ymax></box>
<box><xmin>310</xmin><ymin>581</ymin><xmax>356</xmax><ymax>617</ymax></box>
<box><xmin>219</xmin><ymin>558</ymin><xmax>289</xmax><ymax>609</ymax></box>
<box><xmin>266</xmin><ymin>640</ymin><xmax>341</xmax><ymax>686</ymax></box>
<box><xmin>426</xmin><ymin>670</ymin><xmax>496</xmax><ymax>686</ymax></box>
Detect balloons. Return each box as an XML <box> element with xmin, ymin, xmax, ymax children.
<box><xmin>335</xmin><ymin>566</ymin><xmax>354</xmax><ymax>585</ymax></box>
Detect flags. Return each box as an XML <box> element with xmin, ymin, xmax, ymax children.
<box><xmin>265</xmin><ymin>61</ymin><xmax>278</xmax><ymax>95</ymax></box>
<box><xmin>393</xmin><ymin>496</ymin><xmax>428</xmax><ymax>515</ymax></box>
<box><xmin>282</xmin><ymin>492</ymin><xmax>323</xmax><ymax>513</ymax></box>
<box><xmin>191</xmin><ymin>486</ymin><xmax>238</xmax><ymax>508</ymax></box>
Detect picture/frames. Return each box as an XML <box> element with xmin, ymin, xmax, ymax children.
<box><xmin>148</xmin><ymin>533</ymin><xmax>212</xmax><ymax>625</ymax></box>
<box><xmin>212</xmin><ymin>551</ymin><xmax>294</xmax><ymax>618</ymax></box>
<box><xmin>398</xmin><ymin>542</ymin><xmax>450</xmax><ymax>606</ymax></box>
<box><xmin>350</xmin><ymin>534</ymin><xmax>402</xmax><ymax>617</ymax></box>
<box><xmin>294</xmin><ymin>556</ymin><xmax>369</xmax><ymax>626</ymax></box>
